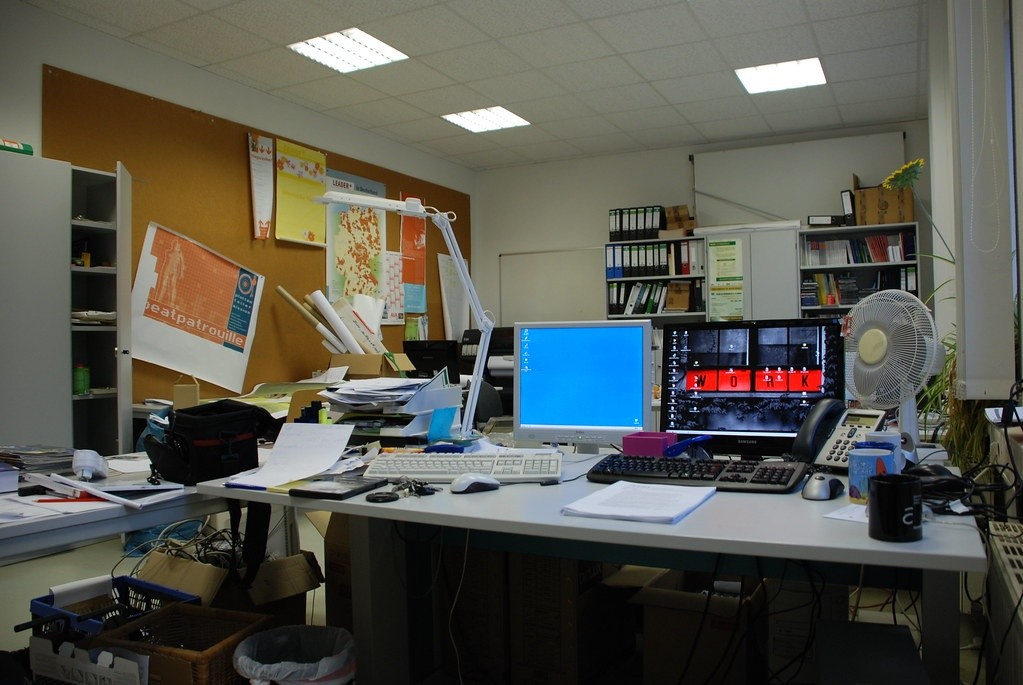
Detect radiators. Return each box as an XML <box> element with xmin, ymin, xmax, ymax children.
<box><xmin>982</xmin><ymin>522</ymin><xmax>1023</xmax><ymax>685</ymax></box>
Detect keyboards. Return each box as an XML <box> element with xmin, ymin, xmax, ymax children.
<box><xmin>365</xmin><ymin>452</ymin><xmax>562</xmax><ymax>484</ymax></box>
<box><xmin>587</xmin><ymin>454</ymin><xmax>808</xmax><ymax>494</ymax></box>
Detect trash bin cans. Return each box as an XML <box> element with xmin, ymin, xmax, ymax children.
<box><xmin>233</xmin><ymin>623</ymin><xmax>355</xmax><ymax>681</ymax></box>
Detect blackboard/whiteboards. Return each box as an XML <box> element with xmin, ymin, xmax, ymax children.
<box><xmin>498</xmin><ymin>246</ymin><xmax>606</xmax><ymax>327</ymax></box>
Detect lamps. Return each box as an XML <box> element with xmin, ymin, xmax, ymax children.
<box><xmin>315</xmin><ymin>191</ymin><xmax>496</xmax><ymax>437</ymax></box>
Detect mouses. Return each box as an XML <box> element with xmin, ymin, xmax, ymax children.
<box><xmin>450</xmin><ymin>473</ymin><xmax>500</xmax><ymax>494</ymax></box>
<box><xmin>801</xmin><ymin>472</ymin><xmax>845</xmax><ymax>501</ymax></box>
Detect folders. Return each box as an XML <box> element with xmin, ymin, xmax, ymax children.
<box><xmin>606</xmin><ymin>205</ymin><xmax>704</xmax><ymax>315</ymax></box>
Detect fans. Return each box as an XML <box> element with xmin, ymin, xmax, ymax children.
<box><xmin>840</xmin><ymin>289</ymin><xmax>949</xmax><ymax>459</ymax></box>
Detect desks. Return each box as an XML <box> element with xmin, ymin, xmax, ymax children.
<box><xmin>0</xmin><ymin>429</ymin><xmax>986</xmax><ymax>685</ymax></box>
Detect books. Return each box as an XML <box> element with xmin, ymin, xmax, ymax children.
<box><xmin>0</xmin><ymin>444</ymin><xmax>77</xmax><ymax>473</ymax></box>
<box><xmin>71</xmin><ymin>311</ymin><xmax>116</xmax><ymax>326</ymax></box>
<box><xmin>802</xmin><ymin>232</ymin><xmax>917</xmax><ymax>267</ymax></box>
<box><xmin>352</xmin><ymin>293</ymin><xmax>386</xmax><ymax>341</ymax></box>
<box><xmin>984</xmin><ymin>406</ymin><xmax>1023</xmax><ymax>426</ymax></box>
<box><xmin>560</xmin><ymin>479</ymin><xmax>717</xmax><ymax>525</ymax></box>
<box><xmin>800</xmin><ymin>267</ymin><xmax>918</xmax><ymax>306</ymax></box>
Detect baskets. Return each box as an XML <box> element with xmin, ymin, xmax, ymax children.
<box><xmin>92</xmin><ymin>604</ymin><xmax>275</xmax><ymax>685</ymax></box>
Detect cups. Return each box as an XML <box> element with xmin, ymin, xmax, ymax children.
<box><xmin>868</xmin><ymin>474</ymin><xmax>923</xmax><ymax>543</ymax></box>
<box><xmin>848</xmin><ymin>448</ymin><xmax>893</xmax><ymax>505</ymax></box>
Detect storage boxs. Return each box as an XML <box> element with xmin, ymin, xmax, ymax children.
<box><xmin>600</xmin><ymin>564</ymin><xmax>783</xmax><ymax>685</ymax></box>
<box><xmin>441</xmin><ymin>544</ymin><xmax>510</xmax><ymax>685</ymax></box>
<box><xmin>330</xmin><ymin>353</ymin><xmax>417</xmax><ymax>380</ymax></box>
<box><xmin>664</xmin><ymin>205</ymin><xmax>695</xmax><ymax>237</ymax></box>
<box><xmin>508</xmin><ymin>552</ymin><xmax>636</xmax><ymax>685</ymax></box>
<box><xmin>29</xmin><ymin>575</ymin><xmax>202</xmax><ymax>664</ymax></box>
<box><xmin>852</xmin><ymin>173</ymin><xmax>917</xmax><ymax>226</ymax></box>
<box><xmin>305</xmin><ymin>511</ymin><xmax>353</xmax><ymax>635</ymax></box>
<box><xmin>666</xmin><ymin>283</ymin><xmax>690</xmax><ymax>309</ymax></box>
<box><xmin>137</xmin><ymin>538</ymin><xmax>326</xmax><ymax>632</ymax></box>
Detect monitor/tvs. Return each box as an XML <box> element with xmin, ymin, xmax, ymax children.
<box><xmin>402</xmin><ymin>340</ymin><xmax>460</xmax><ymax>383</ymax></box>
<box><xmin>660</xmin><ymin>317</ymin><xmax>846</xmax><ymax>462</ymax></box>
<box><xmin>513</xmin><ymin>318</ymin><xmax>652</xmax><ymax>458</ymax></box>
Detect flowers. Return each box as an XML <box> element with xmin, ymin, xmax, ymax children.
<box><xmin>882</xmin><ymin>158</ymin><xmax>955</xmax><ymax>261</ymax></box>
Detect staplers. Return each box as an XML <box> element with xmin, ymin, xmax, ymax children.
<box><xmin>665</xmin><ymin>435</ymin><xmax>714</xmax><ymax>463</ymax></box>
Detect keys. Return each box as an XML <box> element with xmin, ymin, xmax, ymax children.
<box><xmin>393</xmin><ymin>475</ymin><xmax>443</xmax><ymax>498</ymax></box>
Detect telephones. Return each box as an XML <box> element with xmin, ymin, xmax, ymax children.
<box><xmin>791</xmin><ymin>398</ymin><xmax>889</xmax><ymax>474</ymax></box>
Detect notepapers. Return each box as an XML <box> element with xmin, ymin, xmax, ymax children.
<box><xmin>267</xmin><ymin>479</ymin><xmax>310</xmax><ymax>495</ymax></box>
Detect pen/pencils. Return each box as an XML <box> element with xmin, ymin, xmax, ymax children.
<box><xmin>33</xmin><ymin>498</ymin><xmax>108</xmax><ymax>502</ymax></box>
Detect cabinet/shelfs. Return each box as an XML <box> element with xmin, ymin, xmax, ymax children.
<box><xmin>694</xmin><ymin>226</ymin><xmax>800</xmax><ymax>321</ymax></box>
<box><xmin>797</xmin><ymin>221</ymin><xmax>922</xmax><ymax>408</ymax></box>
<box><xmin>603</xmin><ymin>236</ymin><xmax>708</xmax><ymax>322</ymax></box>
<box><xmin>0</xmin><ymin>150</ymin><xmax>133</xmax><ymax>566</ymax></box>
